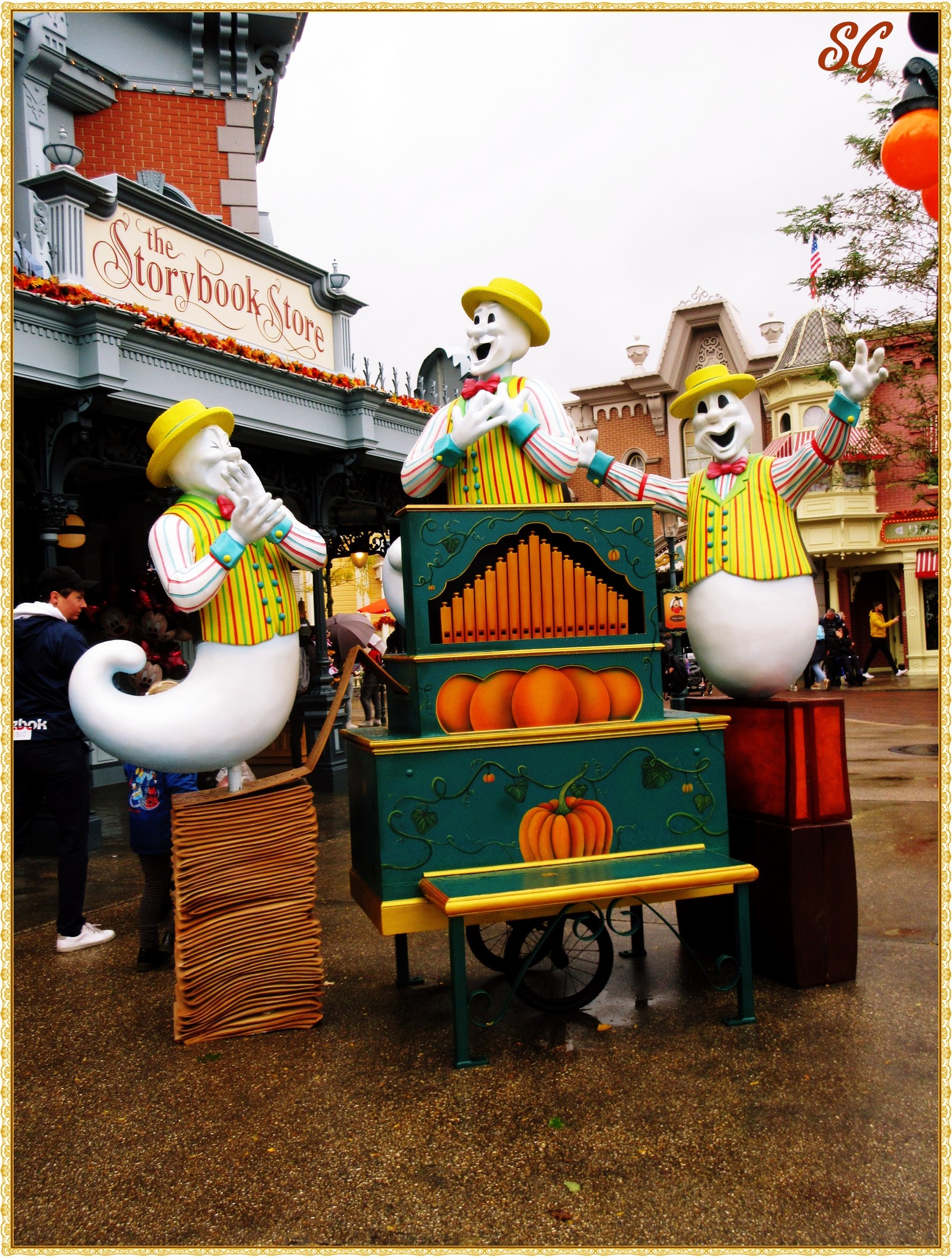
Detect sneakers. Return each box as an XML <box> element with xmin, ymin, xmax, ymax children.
<box><xmin>56</xmin><ymin>922</ymin><xmax>116</xmax><ymax>953</ymax></box>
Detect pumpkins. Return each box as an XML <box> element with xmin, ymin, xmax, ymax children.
<box><xmin>518</xmin><ymin>764</ymin><xmax>613</xmax><ymax>863</ymax></box>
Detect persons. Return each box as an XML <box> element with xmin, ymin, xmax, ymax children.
<box><xmin>125</xmin><ymin>763</ymin><xmax>203</xmax><ymax>970</ymax></box>
<box><xmin>13</xmin><ymin>566</ymin><xmax>118</xmax><ymax>955</ymax></box>
<box><xmin>809</xmin><ymin>608</ymin><xmax>866</xmax><ymax>689</ymax></box>
<box><xmin>367</xmin><ymin>645</ymin><xmax>382</xmax><ymax>667</ymax></box>
<box><xmin>343</xmin><ymin>673</ymin><xmax>358</xmax><ymax>730</ymax></box>
<box><xmin>289</xmin><ymin>692</ymin><xmax>305</xmax><ymax>769</ymax></box>
<box><xmin>862</xmin><ymin>603</ymin><xmax>908</xmax><ymax>679</ymax></box>
<box><xmin>358</xmin><ymin>656</ymin><xmax>382</xmax><ymax>726</ymax></box>
<box><xmin>562</xmin><ymin>338</ymin><xmax>890</xmax><ymax>699</ymax></box>
<box><xmin>400</xmin><ymin>276</ymin><xmax>580</xmax><ymax>505</ymax></box>
<box><xmin>69</xmin><ymin>398</ymin><xmax>328</xmax><ymax>771</ymax></box>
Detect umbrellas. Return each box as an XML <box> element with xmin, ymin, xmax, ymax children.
<box><xmin>368</xmin><ymin>633</ymin><xmax>388</xmax><ymax>655</ymax></box>
<box><xmin>357</xmin><ymin>598</ymin><xmax>391</xmax><ymax>614</ymax></box>
<box><xmin>325</xmin><ymin>613</ymin><xmax>376</xmax><ymax>657</ymax></box>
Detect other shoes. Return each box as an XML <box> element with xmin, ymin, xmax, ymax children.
<box><xmin>357</xmin><ymin>720</ymin><xmax>373</xmax><ymax>727</ymax></box>
<box><xmin>346</xmin><ymin>724</ymin><xmax>358</xmax><ymax>729</ymax></box>
<box><xmin>372</xmin><ymin>719</ymin><xmax>381</xmax><ymax>726</ymax></box>
<box><xmin>790</xmin><ymin>668</ymin><xmax>908</xmax><ymax>691</ymax></box>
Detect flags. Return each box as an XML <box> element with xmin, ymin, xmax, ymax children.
<box><xmin>809</xmin><ymin>232</ymin><xmax>822</xmax><ymax>299</ymax></box>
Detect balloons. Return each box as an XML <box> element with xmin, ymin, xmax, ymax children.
<box><xmin>82</xmin><ymin>589</ymin><xmax>194</xmax><ymax>694</ymax></box>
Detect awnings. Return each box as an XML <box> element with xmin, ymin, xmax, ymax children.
<box><xmin>920</xmin><ymin>414</ymin><xmax>939</xmax><ymax>454</ymax></box>
<box><xmin>840</xmin><ymin>428</ymin><xmax>889</xmax><ymax>463</ymax></box>
<box><xmin>794</xmin><ymin>431</ymin><xmax>818</xmax><ymax>453</ymax></box>
<box><xmin>762</xmin><ymin>434</ymin><xmax>791</xmax><ymax>459</ymax></box>
<box><xmin>914</xmin><ymin>548</ymin><xmax>939</xmax><ymax>579</ymax></box>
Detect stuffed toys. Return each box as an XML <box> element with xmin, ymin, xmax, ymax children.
<box><xmin>326</xmin><ymin>629</ymin><xmax>340</xmax><ymax>686</ymax></box>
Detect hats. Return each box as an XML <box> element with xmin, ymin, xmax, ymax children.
<box><xmin>36</xmin><ymin>565</ymin><xmax>99</xmax><ymax>590</ymax></box>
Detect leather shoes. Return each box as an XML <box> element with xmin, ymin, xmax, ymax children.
<box><xmin>135</xmin><ymin>948</ymin><xmax>176</xmax><ymax>972</ymax></box>
<box><xmin>160</xmin><ymin>932</ymin><xmax>176</xmax><ymax>954</ymax></box>
